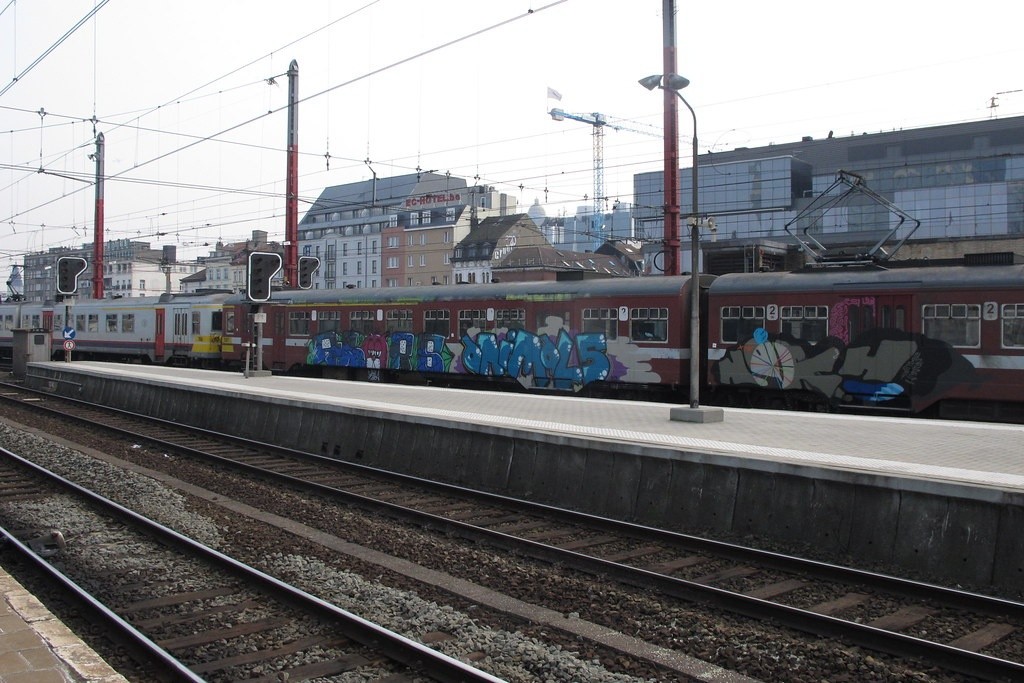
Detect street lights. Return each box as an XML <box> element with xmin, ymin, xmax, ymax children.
<box><xmin>638</xmin><ymin>72</ymin><xmax>700</xmax><ymax>410</ymax></box>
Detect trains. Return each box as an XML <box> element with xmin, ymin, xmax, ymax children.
<box><xmin>3</xmin><ymin>257</ymin><xmax>1024</xmax><ymax>425</ymax></box>
